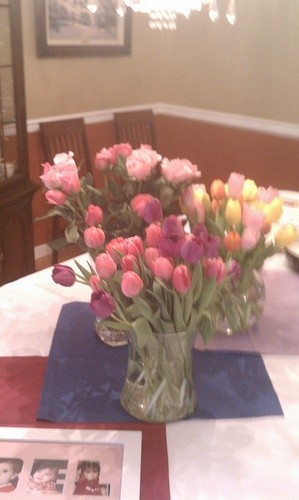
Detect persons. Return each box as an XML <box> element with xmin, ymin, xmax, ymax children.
<box><xmin>73</xmin><ymin>461</ymin><xmax>102</xmax><ymax>495</ymax></box>
<box><xmin>29</xmin><ymin>461</ymin><xmax>60</xmax><ymax>495</ymax></box>
<box><xmin>0</xmin><ymin>458</ymin><xmax>23</xmax><ymax>493</ymax></box>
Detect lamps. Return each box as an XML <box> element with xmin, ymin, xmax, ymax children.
<box><xmin>123</xmin><ymin>0</ymin><xmax>237</xmax><ymax>30</ymax></box>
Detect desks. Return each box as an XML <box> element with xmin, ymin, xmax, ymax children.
<box><xmin>0</xmin><ymin>188</ymin><xmax>299</xmax><ymax>500</ymax></box>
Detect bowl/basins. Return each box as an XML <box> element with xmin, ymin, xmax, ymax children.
<box><xmin>0</xmin><ymin>162</ymin><xmax>15</xmax><ymax>181</ymax></box>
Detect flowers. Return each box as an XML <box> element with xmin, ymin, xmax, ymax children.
<box><xmin>35</xmin><ymin>143</ymin><xmax>285</xmax><ymax>333</ymax></box>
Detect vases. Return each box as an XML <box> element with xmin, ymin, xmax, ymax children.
<box><xmin>93</xmin><ymin>307</ymin><xmax>129</xmax><ymax>349</ymax></box>
<box><xmin>122</xmin><ymin>316</ymin><xmax>204</xmax><ymax>422</ymax></box>
<box><xmin>208</xmin><ymin>269</ymin><xmax>268</xmax><ymax>338</ymax></box>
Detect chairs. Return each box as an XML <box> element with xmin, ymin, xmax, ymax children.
<box><xmin>38</xmin><ymin>116</ymin><xmax>90</xmax><ymax>266</ymax></box>
<box><xmin>113</xmin><ymin>108</ymin><xmax>159</xmax><ymax>168</ymax></box>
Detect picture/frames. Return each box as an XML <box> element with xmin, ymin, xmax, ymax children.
<box><xmin>34</xmin><ymin>0</ymin><xmax>132</xmax><ymax>57</ymax></box>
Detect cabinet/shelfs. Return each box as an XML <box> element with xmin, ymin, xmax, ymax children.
<box><xmin>0</xmin><ymin>0</ymin><xmax>38</xmax><ymax>286</ymax></box>
